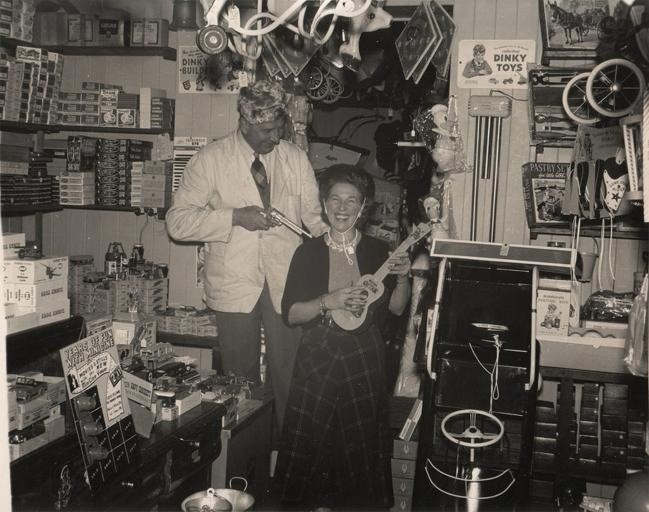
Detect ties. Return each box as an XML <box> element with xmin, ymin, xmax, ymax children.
<box><xmin>250</xmin><ymin>153</ymin><xmax>269</xmax><ymax>208</ymax></box>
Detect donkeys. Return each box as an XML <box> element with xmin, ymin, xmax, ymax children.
<box><xmin>548</xmin><ymin>0</ymin><xmax>584</xmax><ymax>45</ymax></box>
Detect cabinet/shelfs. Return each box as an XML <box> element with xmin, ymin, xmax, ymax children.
<box><xmin>0</xmin><ymin>34</ymin><xmax>176</xmax><ymax>217</ymax></box>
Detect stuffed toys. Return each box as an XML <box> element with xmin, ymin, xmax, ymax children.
<box><xmin>429</xmin><ymin>103</ymin><xmax>460</xmax><ymax>175</ymax></box>
<box><xmin>423</xmin><ymin>198</ymin><xmax>451</xmax><ymax>249</ymax></box>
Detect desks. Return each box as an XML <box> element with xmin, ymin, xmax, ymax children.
<box><xmin>9</xmin><ymin>375</ymin><xmax>275</xmax><ymax>512</ymax></box>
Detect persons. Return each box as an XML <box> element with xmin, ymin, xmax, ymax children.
<box><xmin>272</xmin><ymin>165</ymin><xmax>414</xmax><ymax>509</ymax></box>
<box><xmin>163</xmin><ymin>86</ymin><xmax>330</xmax><ymax>496</ymax></box>
<box><xmin>462</xmin><ymin>44</ymin><xmax>494</xmax><ymax>79</ymax></box>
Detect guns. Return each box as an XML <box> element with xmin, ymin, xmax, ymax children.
<box><xmin>259</xmin><ymin>207</ymin><xmax>314</xmax><ymax>239</ymax></box>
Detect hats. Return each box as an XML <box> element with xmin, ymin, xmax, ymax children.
<box><xmin>237</xmin><ymin>80</ymin><xmax>288</xmax><ymax>125</ymax></box>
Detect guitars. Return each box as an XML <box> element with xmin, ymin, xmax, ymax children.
<box><xmin>331</xmin><ymin>222</ymin><xmax>431</xmax><ymax>331</ymax></box>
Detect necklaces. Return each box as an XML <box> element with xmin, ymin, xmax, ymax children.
<box><xmin>324</xmin><ymin>229</ymin><xmax>363</xmax><ymax>250</ymax></box>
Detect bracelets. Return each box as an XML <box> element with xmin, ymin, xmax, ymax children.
<box><xmin>319</xmin><ymin>293</ymin><xmax>328</xmax><ymax>316</ymax></box>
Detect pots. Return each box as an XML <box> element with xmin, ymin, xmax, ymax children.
<box><xmin>180</xmin><ymin>475</ymin><xmax>256</xmax><ymax>512</ymax></box>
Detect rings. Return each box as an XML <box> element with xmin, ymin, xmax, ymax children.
<box><xmin>345</xmin><ymin>298</ymin><xmax>348</xmax><ymax>305</ymax></box>
